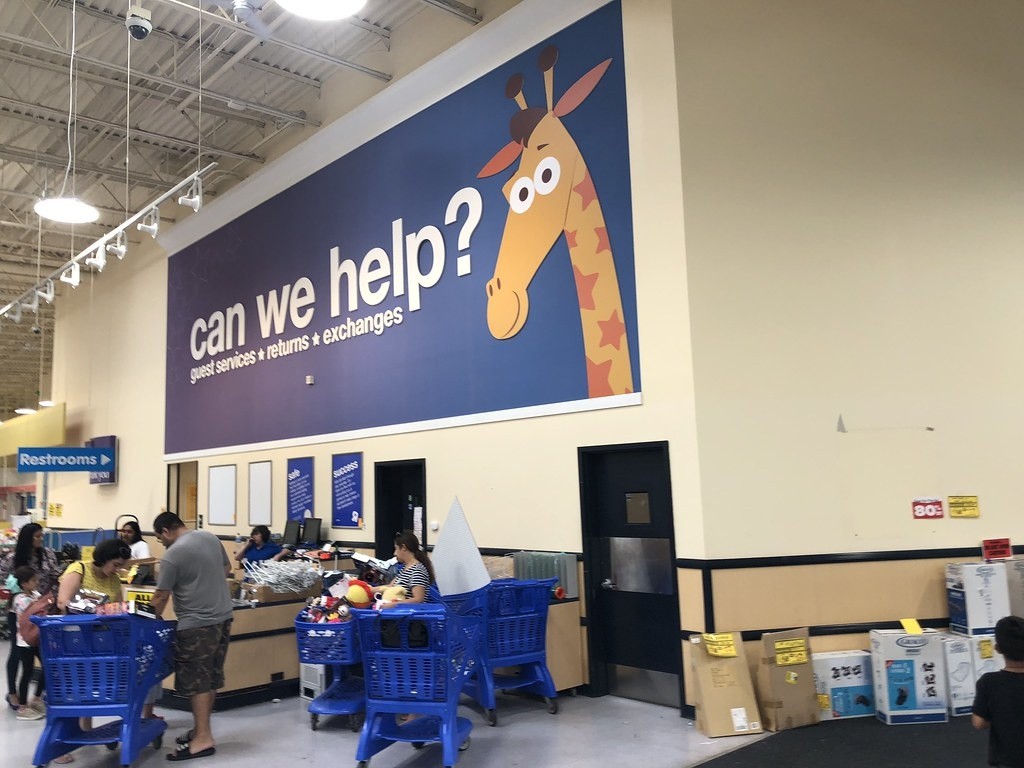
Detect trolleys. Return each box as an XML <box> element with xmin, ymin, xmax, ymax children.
<box><xmin>428</xmin><ymin>575</ymin><xmax>561</xmax><ymax>726</ymax></box>
<box><xmin>27</xmin><ymin>612</ymin><xmax>178</xmax><ymax>768</ymax></box>
<box><xmin>346</xmin><ymin>602</ymin><xmax>486</xmax><ymax>768</ymax></box>
<box><xmin>291</xmin><ymin>581</ymin><xmax>384</xmax><ymax>733</ymax></box>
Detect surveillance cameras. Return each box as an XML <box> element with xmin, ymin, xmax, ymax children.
<box><xmin>32</xmin><ymin>327</ymin><xmax>41</xmax><ymax>334</ymax></box>
<box><xmin>125</xmin><ymin>17</ymin><xmax>152</xmax><ymax>40</ymax></box>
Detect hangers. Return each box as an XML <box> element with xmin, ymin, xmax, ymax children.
<box><xmin>373</xmin><ymin>592</ymin><xmax>382</xmax><ymax>604</ymax></box>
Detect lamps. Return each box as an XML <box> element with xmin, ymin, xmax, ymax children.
<box><xmin>0</xmin><ymin>169</ymin><xmax>203</xmax><ymax>320</ymax></box>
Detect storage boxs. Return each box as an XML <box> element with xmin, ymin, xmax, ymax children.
<box><xmin>241</xmin><ymin>579</ymin><xmax>322</xmax><ymax>602</ymax></box>
<box><xmin>694</xmin><ymin>562</ymin><xmax>1012</xmax><ymax>737</ymax></box>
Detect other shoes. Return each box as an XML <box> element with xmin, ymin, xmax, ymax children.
<box><xmin>5</xmin><ymin>692</ymin><xmax>19</xmax><ymax>710</ymax></box>
<box><xmin>53</xmin><ymin>754</ymin><xmax>74</xmax><ymax>764</ymax></box>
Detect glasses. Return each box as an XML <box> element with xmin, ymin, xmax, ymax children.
<box><xmin>156</xmin><ymin>533</ymin><xmax>163</xmax><ymax>543</ymax></box>
<box><xmin>396</xmin><ymin>530</ymin><xmax>408</xmax><ymax>542</ymax></box>
<box><xmin>121</xmin><ymin>529</ymin><xmax>134</xmax><ymax>533</ymax></box>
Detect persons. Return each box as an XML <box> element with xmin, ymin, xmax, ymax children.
<box><xmin>234</xmin><ymin>525</ymin><xmax>291</xmax><ymax>600</ymax></box>
<box><xmin>370</xmin><ymin>530</ymin><xmax>435</xmax><ymax>724</ymax></box>
<box><xmin>5</xmin><ymin>511</ymin><xmax>234</xmax><ymax>764</ymax></box>
<box><xmin>970</xmin><ymin>616</ymin><xmax>1024</xmax><ymax>768</ymax></box>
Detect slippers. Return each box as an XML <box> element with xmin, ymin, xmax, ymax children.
<box><xmin>165</xmin><ymin>743</ymin><xmax>215</xmax><ymax>761</ymax></box>
<box><xmin>175</xmin><ymin>727</ymin><xmax>196</xmax><ymax>745</ymax></box>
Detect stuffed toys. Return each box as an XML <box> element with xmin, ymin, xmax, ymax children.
<box><xmin>295</xmin><ymin>579</ymin><xmax>408</xmax><ymax>624</ymax></box>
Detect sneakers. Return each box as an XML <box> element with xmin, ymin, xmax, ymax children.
<box><xmin>15</xmin><ymin>704</ymin><xmax>44</xmax><ymax>720</ymax></box>
<box><xmin>30</xmin><ymin>696</ymin><xmax>48</xmax><ymax>716</ymax></box>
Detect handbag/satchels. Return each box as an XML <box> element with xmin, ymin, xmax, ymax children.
<box><xmin>18</xmin><ymin>562</ymin><xmax>85</xmax><ymax>648</ymax></box>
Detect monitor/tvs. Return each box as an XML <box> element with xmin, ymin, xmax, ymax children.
<box><xmin>302</xmin><ymin>518</ymin><xmax>322</xmax><ymax>545</ymax></box>
<box><xmin>283</xmin><ymin>520</ymin><xmax>300</xmax><ymax>545</ymax></box>
